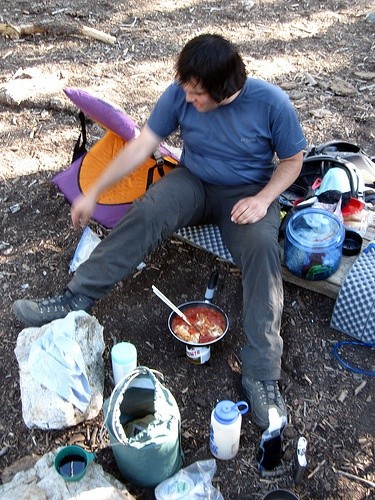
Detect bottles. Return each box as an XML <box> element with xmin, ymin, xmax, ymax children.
<box><xmin>111</xmin><ymin>342</ymin><xmax>137</xmax><ymax>386</ymax></box>
<box><xmin>210</xmin><ymin>400</ymin><xmax>248</xmax><ymax>460</ymax></box>
<box><xmin>284</xmin><ymin>210</ymin><xmax>345</xmax><ymax>280</ymax></box>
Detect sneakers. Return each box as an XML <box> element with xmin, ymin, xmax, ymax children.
<box><xmin>242</xmin><ymin>376</ymin><xmax>287</xmax><ymax>429</ymax></box>
<box><xmin>12</xmin><ymin>287</ymin><xmax>93</xmax><ymax>327</ymax></box>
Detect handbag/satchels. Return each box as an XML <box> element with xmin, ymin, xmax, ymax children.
<box><xmin>103</xmin><ymin>366</ymin><xmax>185</xmax><ymax>485</ymax></box>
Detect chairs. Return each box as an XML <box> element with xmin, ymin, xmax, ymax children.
<box><xmin>50</xmin><ymin>89</ymin><xmax>183</xmax><ymax>232</ymax></box>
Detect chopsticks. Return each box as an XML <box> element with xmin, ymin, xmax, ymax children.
<box><xmin>152</xmin><ymin>284</ymin><xmax>191</xmax><ymax>326</ymax></box>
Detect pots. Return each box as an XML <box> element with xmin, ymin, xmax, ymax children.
<box><xmin>167</xmin><ymin>301</ymin><xmax>229</xmax><ymax>346</ymax></box>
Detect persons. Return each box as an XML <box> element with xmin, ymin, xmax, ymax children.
<box><xmin>12</xmin><ymin>33</ymin><xmax>308</xmax><ymax>431</ymax></box>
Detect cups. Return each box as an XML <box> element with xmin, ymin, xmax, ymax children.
<box><xmin>55</xmin><ymin>446</ymin><xmax>94</xmax><ymax>481</ymax></box>
